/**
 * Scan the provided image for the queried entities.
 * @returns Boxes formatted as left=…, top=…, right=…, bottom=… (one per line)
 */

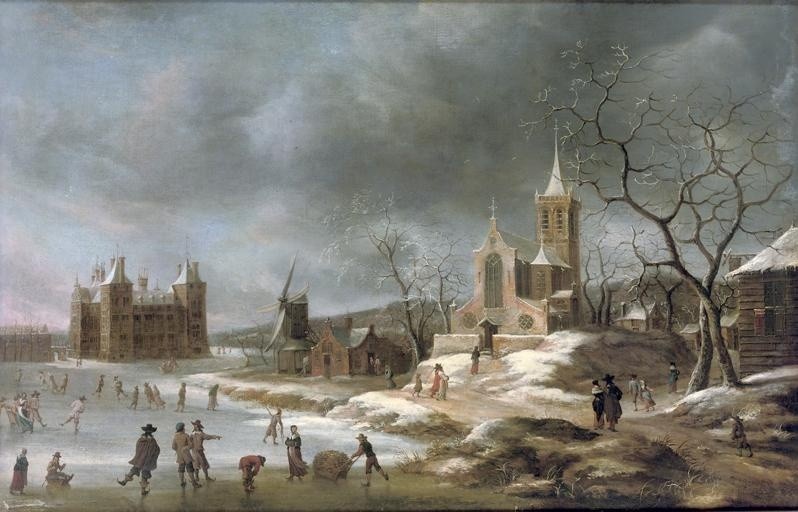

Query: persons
left=412, top=373, right=422, bottom=397
left=470, top=346, right=481, bottom=375
left=591, top=380, right=605, bottom=430
left=429, top=363, right=442, bottom=397
left=628, top=374, right=641, bottom=411
left=436, top=366, right=449, bottom=401
left=602, top=373, right=623, bottom=432
left=668, top=361, right=677, bottom=394
left=0, top=345, right=397, bottom=495
left=730, top=415, right=753, bottom=457
left=639, top=379, right=656, bottom=412
